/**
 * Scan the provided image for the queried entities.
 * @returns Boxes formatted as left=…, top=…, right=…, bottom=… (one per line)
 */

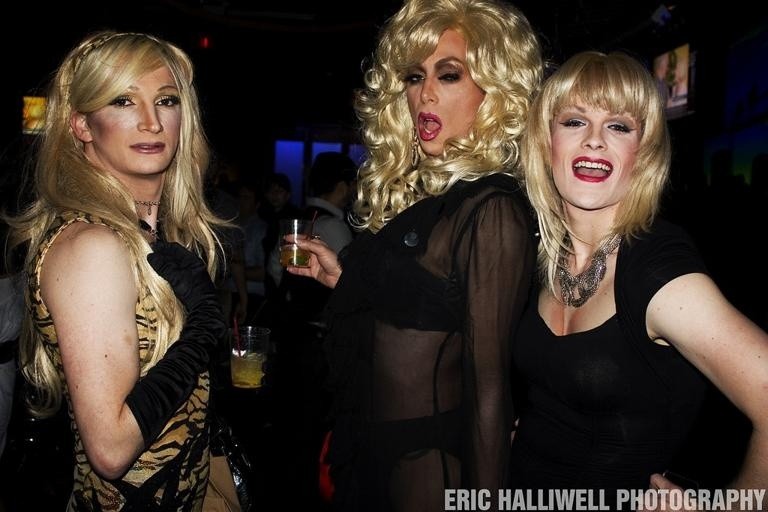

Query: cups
left=278, top=220, right=311, bottom=269
left=230, top=324, right=269, bottom=390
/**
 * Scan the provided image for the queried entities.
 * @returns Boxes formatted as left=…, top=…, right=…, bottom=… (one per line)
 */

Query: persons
left=2, top=0, right=767, bottom=512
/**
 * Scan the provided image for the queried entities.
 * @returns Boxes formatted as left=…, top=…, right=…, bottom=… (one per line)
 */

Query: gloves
left=122, top=240, right=233, bottom=453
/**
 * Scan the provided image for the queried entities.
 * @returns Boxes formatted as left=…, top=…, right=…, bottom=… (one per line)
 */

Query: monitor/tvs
left=652, top=41, right=696, bottom=120
